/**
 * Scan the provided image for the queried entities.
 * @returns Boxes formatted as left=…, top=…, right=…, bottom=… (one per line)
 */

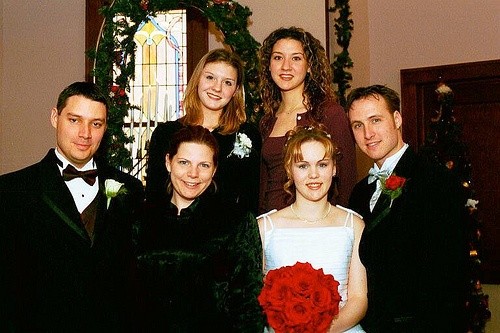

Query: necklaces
left=291, top=202, right=332, bottom=222
left=280, top=99, right=303, bottom=114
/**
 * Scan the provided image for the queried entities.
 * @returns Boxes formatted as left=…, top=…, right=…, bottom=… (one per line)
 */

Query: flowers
left=105, top=178, right=128, bottom=210
left=226, top=131, right=254, bottom=159
left=258, top=263, right=342, bottom=333
left=378, top=172, right=407, bottom=209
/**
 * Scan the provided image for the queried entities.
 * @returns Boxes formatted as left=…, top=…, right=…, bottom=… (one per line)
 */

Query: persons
left=147, top=49, right=262, bottom=223
left=256, top=124, right=368, bottom=333
left=119, top=126, right=262, bottom=333
left=348, top=85, right=486, bottom=333
left=258, top=27, right=359, bottom=208
left=0, top=81, right=146, bottom=333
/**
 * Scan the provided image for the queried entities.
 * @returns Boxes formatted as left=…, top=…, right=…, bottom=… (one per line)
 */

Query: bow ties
left=368, top=168, right=389, bottom=185
left=56, top=157, right=98, bottom=186
